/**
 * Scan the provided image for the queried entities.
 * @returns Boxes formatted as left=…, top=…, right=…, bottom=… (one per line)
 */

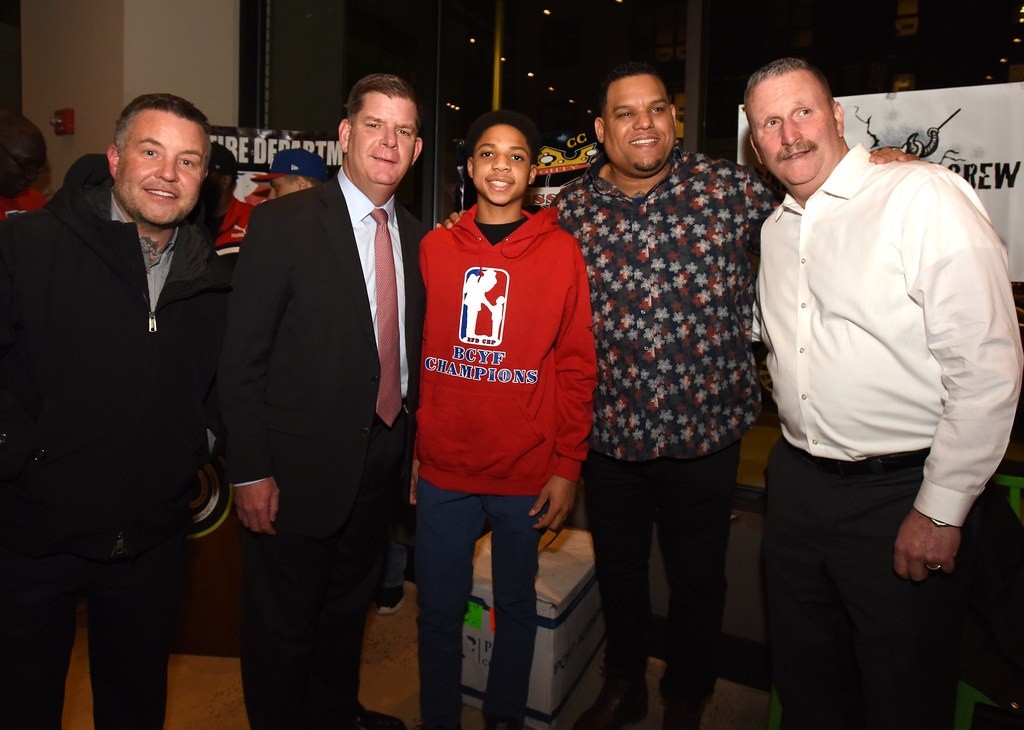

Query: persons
left=744, top=58, right=1023, bottom=730
left=251, top=147, right=407, bottom=614
left=412, top=111, right=597, bottom=730
left=207, top=74, right=428, bottom=730
left=0, top=108, right=255, bottom=272
left=433, top=62, right=919, bottom=730
left=0, top=93, right=232, bottom=730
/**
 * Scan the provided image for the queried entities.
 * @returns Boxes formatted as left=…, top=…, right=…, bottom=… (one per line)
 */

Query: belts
left=372, top=398, right=408, bottom=425
left=780, top=432, right=931, bottom=475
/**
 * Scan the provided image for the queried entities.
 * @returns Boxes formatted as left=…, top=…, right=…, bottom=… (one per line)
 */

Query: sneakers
left=373, top=584, right=405, bottom=615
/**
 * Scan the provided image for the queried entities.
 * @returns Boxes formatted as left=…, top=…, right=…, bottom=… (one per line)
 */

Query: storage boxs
left=461, top=529, right=610, bottom=730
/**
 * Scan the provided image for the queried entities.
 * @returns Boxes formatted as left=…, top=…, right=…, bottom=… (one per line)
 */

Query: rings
left=926, top=565, right=940, bottom=571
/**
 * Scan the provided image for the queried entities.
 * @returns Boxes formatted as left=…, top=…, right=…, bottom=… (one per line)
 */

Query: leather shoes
left=342, top=710, right=407, bottom=730
left=572, top=662, right=648, bottom=730
left=662, top=696, right=705, bottom=730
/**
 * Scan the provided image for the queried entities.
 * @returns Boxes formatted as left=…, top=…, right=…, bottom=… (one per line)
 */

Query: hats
left=252, top=148, right=328, bottom=183
left=208, top=141, right=237, bottom=172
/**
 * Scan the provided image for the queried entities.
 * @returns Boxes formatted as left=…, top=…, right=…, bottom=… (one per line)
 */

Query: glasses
left=0, top=144, right=46, bottom=172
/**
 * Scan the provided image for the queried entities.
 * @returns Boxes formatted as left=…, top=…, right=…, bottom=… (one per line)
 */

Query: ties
left=369, top=209, right=403, bottom=428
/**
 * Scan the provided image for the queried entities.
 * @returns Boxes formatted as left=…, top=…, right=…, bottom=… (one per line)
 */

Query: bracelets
left=931, top=519, right=946, bottom=526
